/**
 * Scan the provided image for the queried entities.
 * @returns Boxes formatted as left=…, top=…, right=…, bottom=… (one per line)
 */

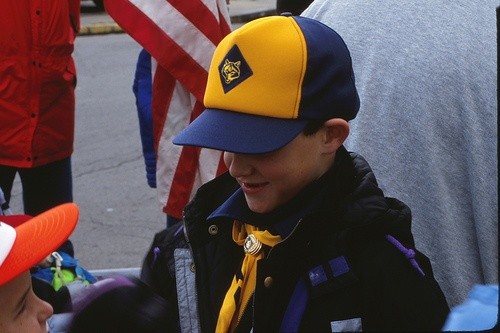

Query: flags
left=103, top=0, right=234, bottom=227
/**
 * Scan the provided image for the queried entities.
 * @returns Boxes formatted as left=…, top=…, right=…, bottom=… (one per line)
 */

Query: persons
left=139, top=12, right=450, bottom=333
left=0, top=203, right=79, bottom=333
left=0, top=0, right=80, bottom=258
left=300, top=0, right=500, bottom=307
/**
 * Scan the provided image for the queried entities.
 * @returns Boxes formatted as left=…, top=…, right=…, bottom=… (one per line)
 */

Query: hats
left=172, top=12, right=360, bottom=153
left=0, top=203, right=78, bottom=286
